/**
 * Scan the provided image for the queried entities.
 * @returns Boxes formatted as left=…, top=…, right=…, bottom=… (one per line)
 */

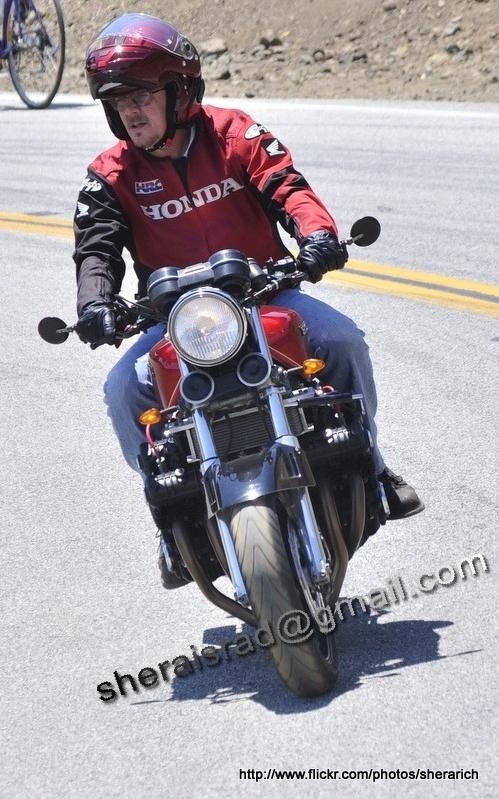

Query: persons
left=71, top=11, right=427, bottom=592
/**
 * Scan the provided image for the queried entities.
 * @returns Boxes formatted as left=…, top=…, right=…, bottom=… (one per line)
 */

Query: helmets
left=83, top=12, right=205, bottom=140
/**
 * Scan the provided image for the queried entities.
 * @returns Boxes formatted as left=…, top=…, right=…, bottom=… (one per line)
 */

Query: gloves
left=296, top=233, right=345, bottom=284
left=76, top=300, right=117, bottom=345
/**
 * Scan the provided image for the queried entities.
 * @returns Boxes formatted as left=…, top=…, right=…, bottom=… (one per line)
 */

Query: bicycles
left=1, top=0, right=68, bottom=112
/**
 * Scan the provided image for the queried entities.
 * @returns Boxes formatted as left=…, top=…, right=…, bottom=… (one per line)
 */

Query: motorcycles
left=37, top=217, right=381, bottom=698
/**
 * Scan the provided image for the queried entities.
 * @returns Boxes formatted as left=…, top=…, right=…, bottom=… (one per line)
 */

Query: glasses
left=109, top=87, right=167, bottom=112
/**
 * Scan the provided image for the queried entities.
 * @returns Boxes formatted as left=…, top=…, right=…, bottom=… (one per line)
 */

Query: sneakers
left=14, top=9, right=46, bottom=22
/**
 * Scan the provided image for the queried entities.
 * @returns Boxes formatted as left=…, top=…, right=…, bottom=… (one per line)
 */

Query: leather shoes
left=377, top=466, right=420, bottom=514
left=155, top=529, right=188, bottom=584
